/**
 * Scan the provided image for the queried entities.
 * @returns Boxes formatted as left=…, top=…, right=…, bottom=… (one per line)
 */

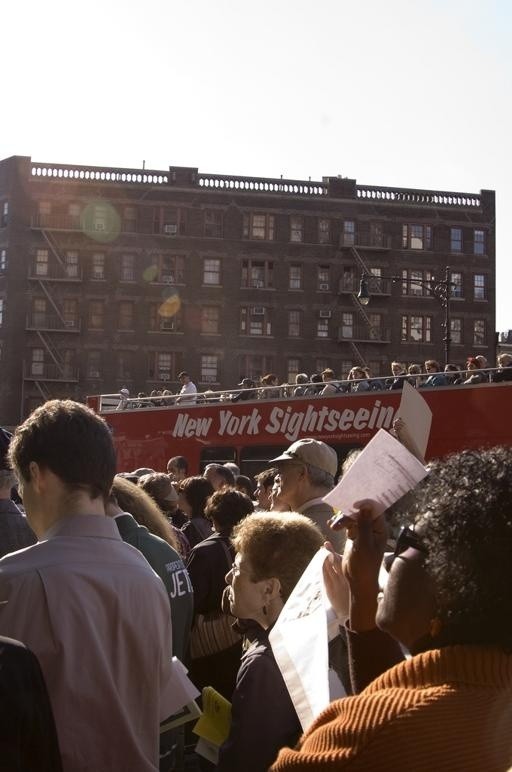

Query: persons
left=137, top=471, right=178, bottom=513
left=252, top=467, right=277, bottom=511
left=0, top=427, right=38, bottom=560
left=164, top=455, right=189, bottom=480
left=424, top=360, right=445, bottom=386
left=346, top=366, right=371, bottom=393
left=149, top=389, right=161, bottom=406
left=202, top=464, right=235, bottom=491
left=408, top=364, right=423, bottom=387
left=320, top=367, right=341, bottom=394
left=104, top=491, right=195, bottom=772
left=176, top=371, right=198, bottom=405
left=493, top=354, right=512, bottom=383
left=218, top=510, right=324, bottom=771
left=268, top=445, right=512, bottom=772
left=268, top=473, right=291, bottom=512
left=178, top=476, right=215, bottom=549
left=269, top=437, right=348, bottom=555
left=163, top=389, right=173, bottom=406
left=0, top=634, right=64, bottom=772
left=258, top=374, right=283, bottom=400
left=310, top=373, right=323, bottom=394
left=117, top=388, right=131, bottom=410
left=293, top=373, right=308, bottom=394
left=443, top=363, right=460, bottom=384
left=137, top=392, right=147, bottom=407
left=389, top=361, right=409, bottom=388
left=0, top=398, right=175, bottom=772
left=231, top=377, right=257, bottom=403
left=392, top=416, right=444, bottom=471
left=234, top=474, right=253, bottom=496
left=187, top=489, right=255, bottom=690
left=460, top=357, right=487, bottom=385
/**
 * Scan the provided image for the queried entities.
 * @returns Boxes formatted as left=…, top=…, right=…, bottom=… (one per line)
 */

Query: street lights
left=357, top=266, right=457, bottom=365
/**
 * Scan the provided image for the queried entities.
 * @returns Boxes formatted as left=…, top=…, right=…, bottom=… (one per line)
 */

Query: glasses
left=394, top=527, right=432, bottom=562
left=228, top=562, right=269, bottom=581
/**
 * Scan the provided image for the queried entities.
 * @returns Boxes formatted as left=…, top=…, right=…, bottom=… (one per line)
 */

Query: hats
left=1, top=427, right=17, bottom=471
left=118, top=387, right=131, bottom=396
left=267, top=436, right=338, bottom=477
left=177, top=371, right=190, bottom=378
left=237, top=378, right=253, bottom=386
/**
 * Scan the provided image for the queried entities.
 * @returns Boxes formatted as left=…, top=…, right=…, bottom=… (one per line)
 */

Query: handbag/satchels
left=188, top=539, right=244, bottom=662
